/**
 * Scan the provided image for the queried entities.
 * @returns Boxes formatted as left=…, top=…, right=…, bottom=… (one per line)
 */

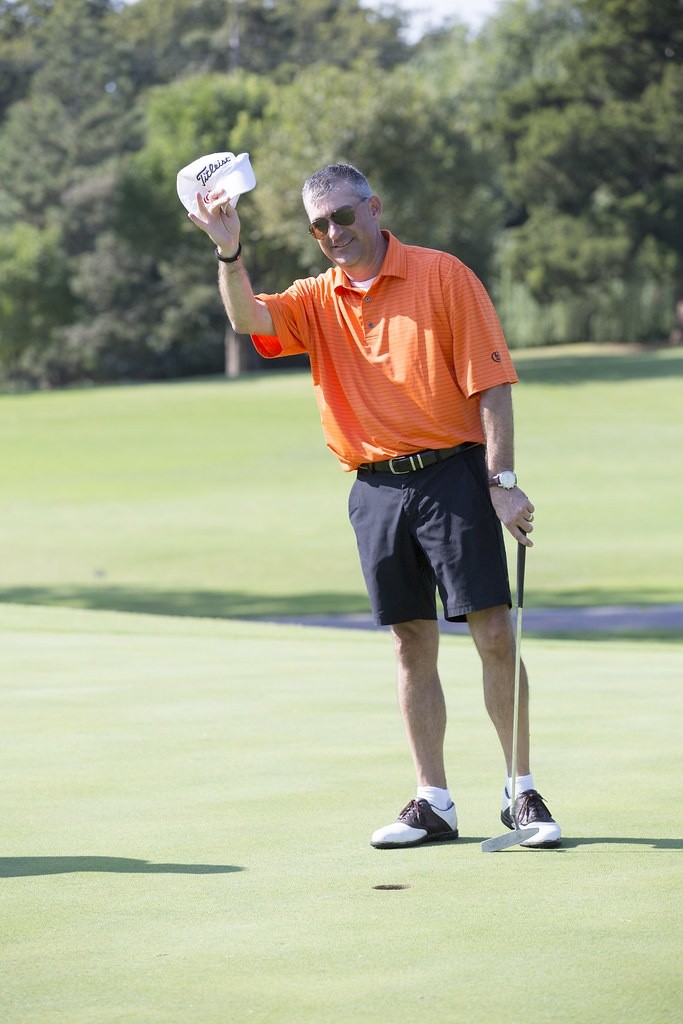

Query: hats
left=175, top=152, right=256, bottom=221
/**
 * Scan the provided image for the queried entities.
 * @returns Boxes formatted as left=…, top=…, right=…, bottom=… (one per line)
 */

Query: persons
left=188, top=163, right=560, bottom=848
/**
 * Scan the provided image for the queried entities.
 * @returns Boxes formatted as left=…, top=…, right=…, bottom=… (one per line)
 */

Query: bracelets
left=215, top=242, right=242, bottom=263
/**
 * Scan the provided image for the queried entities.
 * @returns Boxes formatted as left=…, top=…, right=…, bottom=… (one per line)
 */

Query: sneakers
left=501, top=786, right=562, bottom=848
left=369, top=796, right=459, bottom=849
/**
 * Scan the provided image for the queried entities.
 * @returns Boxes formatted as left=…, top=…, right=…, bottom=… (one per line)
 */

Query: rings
left=527, top=513, right=532, bottom=520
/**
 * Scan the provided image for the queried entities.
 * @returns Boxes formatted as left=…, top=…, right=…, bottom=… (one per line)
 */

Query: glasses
left=307, top=196, right=368, bottom=240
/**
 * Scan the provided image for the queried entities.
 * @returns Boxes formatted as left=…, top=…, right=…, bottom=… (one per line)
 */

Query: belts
left=358, top=441, right=481, bottom=475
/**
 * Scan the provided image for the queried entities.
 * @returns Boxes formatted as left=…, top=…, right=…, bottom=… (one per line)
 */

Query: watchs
left=488, top=471, right=518, bottom=490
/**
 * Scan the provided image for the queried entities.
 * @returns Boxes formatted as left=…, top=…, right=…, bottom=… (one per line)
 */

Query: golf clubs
left=480, top=525, right=540, bottom=853
